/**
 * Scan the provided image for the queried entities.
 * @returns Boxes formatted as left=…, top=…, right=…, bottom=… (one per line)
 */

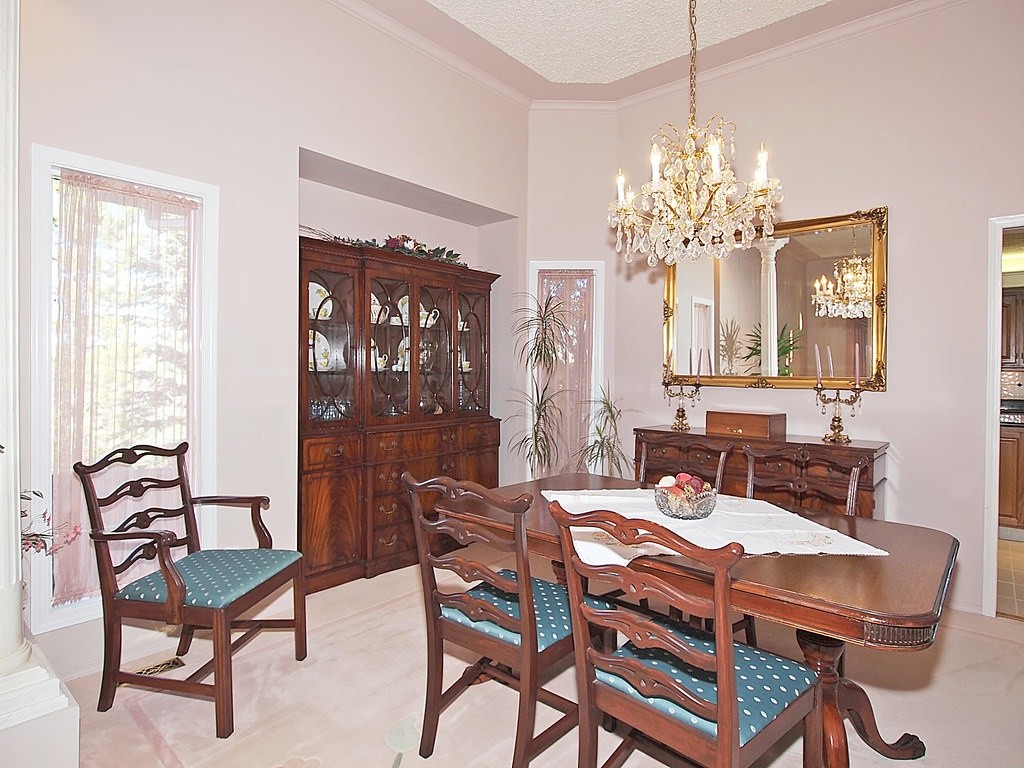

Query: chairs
left=401, top=434, right=869, bottom=768
left=73, top=442, right=307, bottom=738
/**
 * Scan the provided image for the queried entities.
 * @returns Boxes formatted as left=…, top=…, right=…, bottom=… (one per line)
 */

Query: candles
left=666, top=350, right=672, bottom=382
left=696, top=349, right=702, bottom=384
left=690, top=349, right=692, bottom=375
left=855, top=343, right=860, bottom=386
left=708, top=349, right=712, bottom=376
left=827, top=346, right=834, bottom=378
left=814, top=344, right=822, bottom=388
left=866, top=345, right=872, bottom=377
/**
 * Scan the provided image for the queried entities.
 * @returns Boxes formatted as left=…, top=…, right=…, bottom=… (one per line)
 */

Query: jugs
left=371, top=299, right=389, bottom=324
left=420, top=309, right=439, bottom=328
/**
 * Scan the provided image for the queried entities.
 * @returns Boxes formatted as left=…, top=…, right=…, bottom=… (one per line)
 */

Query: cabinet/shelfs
left=1002, top=287, right=1024, bottom=372
left=998, top=424, right=1024, bottom=542
left=298, top=235, right=502, bottom=595
left=633, top=425, right=890, bottom=521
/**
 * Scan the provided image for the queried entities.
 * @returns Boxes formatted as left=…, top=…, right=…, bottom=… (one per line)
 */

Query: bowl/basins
left=657, top=486, right=718, bottom=521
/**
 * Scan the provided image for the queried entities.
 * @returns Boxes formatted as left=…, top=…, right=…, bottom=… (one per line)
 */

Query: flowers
left=20, top=489, right=82, bottom=560
left=299, top=225, right=467, bottom=267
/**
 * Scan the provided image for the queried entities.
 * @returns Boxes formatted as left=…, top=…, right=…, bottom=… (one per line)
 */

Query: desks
left=434, top=472, right=960, bottom=768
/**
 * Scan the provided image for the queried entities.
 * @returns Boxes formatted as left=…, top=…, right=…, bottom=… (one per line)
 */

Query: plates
left=458, top=368, right=473, bottom=372
left=309, top=281, right=333, bottom=321
left=371, top=293, right=380, bottom=305
left=371, top=338, right=389, bottom=371
left=397, top=295, right=424, bottom=314
left=309, top=330, right=333, bottom=371
left=398, top=337, right=409, bottom=363
left=458, top=309, right=470, bottom=331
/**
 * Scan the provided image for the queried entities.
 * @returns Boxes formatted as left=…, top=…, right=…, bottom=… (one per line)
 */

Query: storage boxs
left=706, top=411, right=786, bottom=438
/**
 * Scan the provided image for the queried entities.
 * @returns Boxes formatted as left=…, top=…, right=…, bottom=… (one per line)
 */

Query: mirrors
left=663, top=207, right=889, bottom=392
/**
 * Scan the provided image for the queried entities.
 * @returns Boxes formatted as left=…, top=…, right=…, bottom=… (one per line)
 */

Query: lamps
left=607, top=0, right=784, bottom=267
left=810, top=230, right=872, bottom=319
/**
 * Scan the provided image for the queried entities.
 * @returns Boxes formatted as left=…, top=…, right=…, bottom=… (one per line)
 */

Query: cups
left=459, top=322, right=468, bottom=328
left=462, top=362, right=470, bottom=368
left=390, top=315, right=402, bottom=327
left=377, top=354, right=388, bottom=368
left=316, top=359, right=328, bottom=366
left=313, top=308, right=329, bottom=317
